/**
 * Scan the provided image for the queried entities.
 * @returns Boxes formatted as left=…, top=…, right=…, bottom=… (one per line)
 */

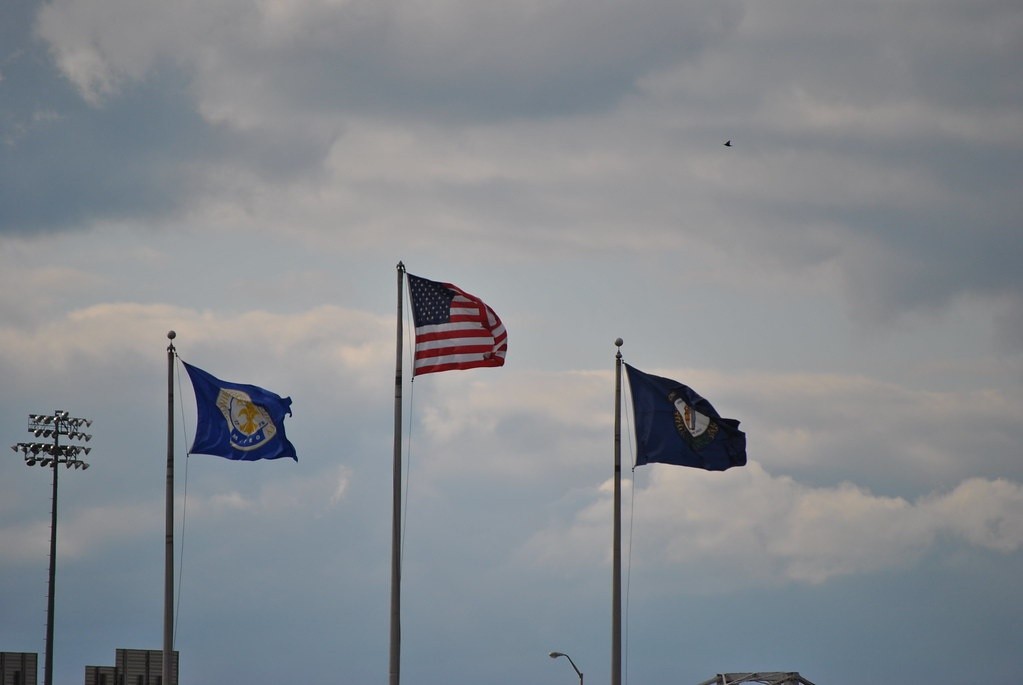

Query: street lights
left=12, top=411, right=92, bottom=685
left=548, top=652, right=583, bottom=685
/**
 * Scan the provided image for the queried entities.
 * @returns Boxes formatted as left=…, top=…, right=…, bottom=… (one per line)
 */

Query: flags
left=624, top=362, right=747, bottom=470
left=406, top=273, right=507, bottom=376
left=182, top=360, right=299, bottom=463
